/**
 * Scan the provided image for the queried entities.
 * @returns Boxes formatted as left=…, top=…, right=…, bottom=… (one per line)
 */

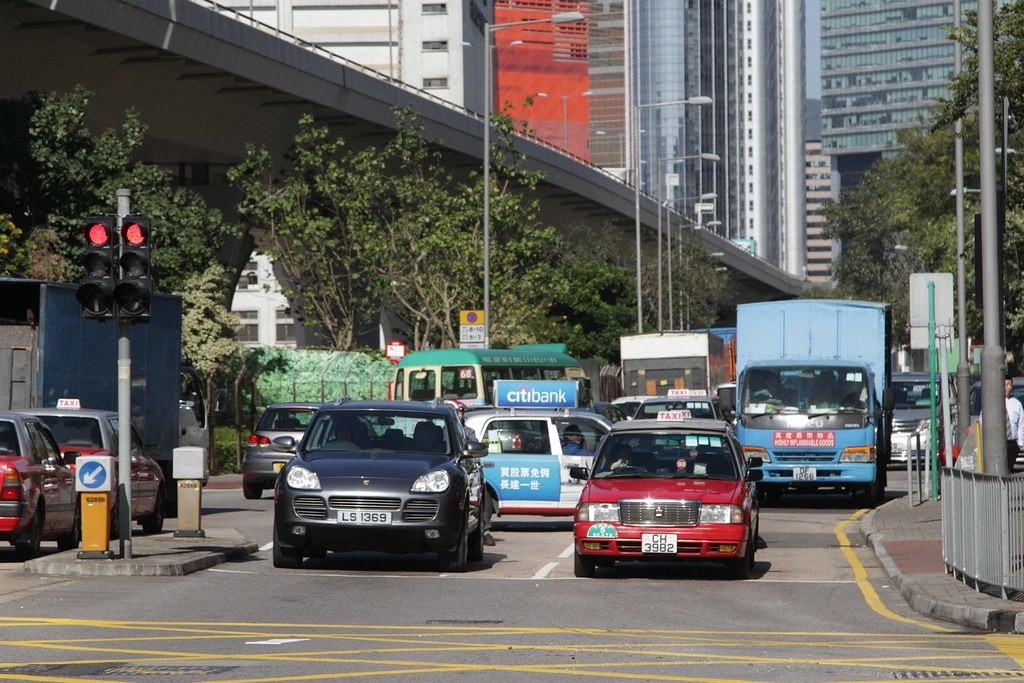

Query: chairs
left=341, top=416, right=363, bottom=443
left=921, top=388, right=930, bottom=399
left=380, top=428, right=405, bottom=448
left=275, top=411, right=295, bottom=427
left=411, top=421, right=436, bottom=445
left=414, top=425, right=448, bottom=451
left=897, top=389, right=908, bottom=401
left=687, top=444, right=729, bottom=471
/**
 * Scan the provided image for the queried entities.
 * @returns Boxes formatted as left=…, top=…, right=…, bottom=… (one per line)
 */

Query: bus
left=391, top=342, right=592, bottom=436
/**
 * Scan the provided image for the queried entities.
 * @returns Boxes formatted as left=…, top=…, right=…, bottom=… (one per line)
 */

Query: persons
left=562, top=425, right=596, bottom=455
left=980, top=374, right=1024, bottom=475
left=442, top=407, right=478, bottom=449
left=597, top=443, right=637, bottom=474
left=749, top=372, right=786, bottom=405
left=801, top=367, right=880, bottom=411
left=325, top=422, right=365, bottom=451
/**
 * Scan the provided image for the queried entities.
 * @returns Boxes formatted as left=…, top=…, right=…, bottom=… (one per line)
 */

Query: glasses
left=576, top=435, right=580, bottom=437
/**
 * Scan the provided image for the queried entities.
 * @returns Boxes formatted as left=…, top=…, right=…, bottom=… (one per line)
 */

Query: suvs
left=273, top=399, right=490, bottom=567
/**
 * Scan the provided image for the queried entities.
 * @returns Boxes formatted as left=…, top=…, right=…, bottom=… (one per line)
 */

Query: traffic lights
left=77, top=215, right=153, bottom=324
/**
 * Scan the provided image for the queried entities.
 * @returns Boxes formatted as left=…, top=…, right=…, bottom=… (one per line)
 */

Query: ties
left=1006, top=409, right=1012, bottom=440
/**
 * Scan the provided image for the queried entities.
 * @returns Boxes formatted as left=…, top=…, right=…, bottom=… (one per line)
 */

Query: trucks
left=0, top=276, right=213, bottom=515
left=729, top=298, right=894, bottom=500
left=619, top=330, right=725, bottom=414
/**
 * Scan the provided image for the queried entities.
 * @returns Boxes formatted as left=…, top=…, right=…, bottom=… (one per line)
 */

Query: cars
left=592, top=393, right=734, bottom=449
left=969, top=380, right=1024, bottom=419
left=890, top=372, right=958, bottom=469
left=0, top=397, right=165, bottom=562
left=462, top=407, right=631, bottom=521
left=242, top=404, right=334, bottom=498
left=570, top=416, right=764, bottom=574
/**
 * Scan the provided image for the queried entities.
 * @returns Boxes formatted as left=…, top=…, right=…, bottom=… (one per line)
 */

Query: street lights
left=537, top=90, right=592, bottom=151
left=597, top=129, right=645, bottom=181
left=458, top=12, right=588, bottom=344
left=631, top=98, right=729, bottom=332
